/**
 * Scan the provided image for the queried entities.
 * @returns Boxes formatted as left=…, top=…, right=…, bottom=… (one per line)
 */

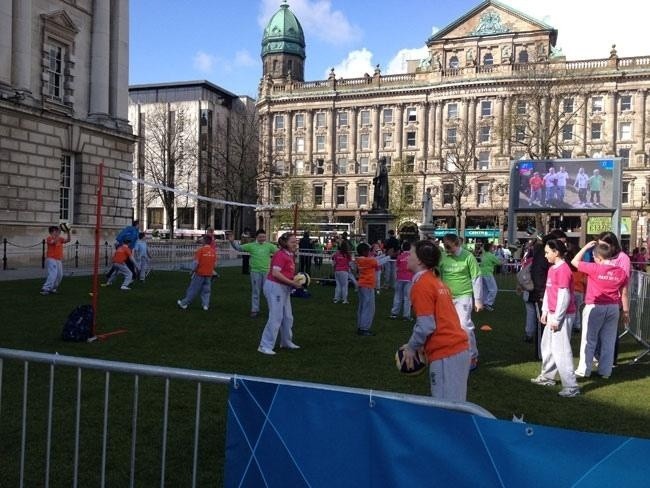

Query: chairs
left=121, top=286, right=130, bottom=289
left=203, top=305, right=208, bottom=311
left=257, top=346, right=276, bottom=356
left=280, top=340, right=300, bottom=350
left=333, top=298, right=413, bottom=337
left=470, top=304, right=609, bottom=398
left=177, top=300, right=188, bottom=309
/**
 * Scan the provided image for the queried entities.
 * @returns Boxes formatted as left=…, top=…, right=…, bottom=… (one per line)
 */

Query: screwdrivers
left=293, top=272, right=310, bottom=288
left=395, top=344, right=427, bottom=375
left=60, top=222, right=71, bottom=231
left=375, top=248, right=382, bottom=255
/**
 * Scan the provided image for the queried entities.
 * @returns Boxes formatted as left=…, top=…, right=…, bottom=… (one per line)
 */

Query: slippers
left=61, top=305, right=93, bottom=342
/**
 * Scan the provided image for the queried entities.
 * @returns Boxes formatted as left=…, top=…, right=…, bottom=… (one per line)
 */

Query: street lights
left=517, top=261, right=534, bottom=291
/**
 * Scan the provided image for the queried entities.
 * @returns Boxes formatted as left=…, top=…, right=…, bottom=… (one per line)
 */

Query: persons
left=461, top=234, right=515, bottom=312
left=202, top=227, right=219, bottom=283
left=240, top=227, right=251, bottom=275
left=257, top=231, right=301, bottom=355
left=103, top=220, right=154, bottom=290
left=569, top=239, right=630, bottom=382
left=519, top=230, right=633, bottom=371
left=298, top=228, right=413, bottom=336
left=439, top=233, right=483, bottom=373
left=530, top=240, right=581, bottom=399
left=517, top=163, right=605, bottom=208
left=178, top=236, right=215, bottom=311
left=421, top=188, right=433, bottom=224
left=227, top=229, right=280, bottom=317
left=633, top=238, right=650, bottom=271
left=372, top=155, right=390, bottom=212
left=399, top=239, right=470, bottom=404
left=41, top=224, right=71, bottom=296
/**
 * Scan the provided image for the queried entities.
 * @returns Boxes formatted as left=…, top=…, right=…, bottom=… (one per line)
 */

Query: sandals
left=143, top=227, right=234, bottom=241
left=276, top=222, right=353, bottom=245
